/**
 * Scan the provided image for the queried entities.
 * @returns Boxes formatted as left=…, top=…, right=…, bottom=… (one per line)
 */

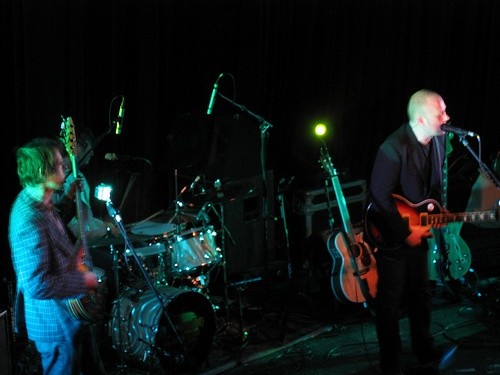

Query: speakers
left=0, top=309, right=28, bottom=375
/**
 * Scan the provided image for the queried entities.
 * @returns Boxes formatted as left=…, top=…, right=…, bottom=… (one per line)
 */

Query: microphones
left=190, top=174, right=203, bottom=188
left=105, top=152, right=144, bottom=161
left=115, top=97, right=125, bottom=135
left=440, top=124, right=478, bottom=137
left=207, top=72, right=224, bottom=115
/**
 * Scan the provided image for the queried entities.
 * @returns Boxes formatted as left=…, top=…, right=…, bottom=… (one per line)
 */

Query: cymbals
left=86, top=234, right=148, bottom=248
left=188, top=176, right=263, bottom=207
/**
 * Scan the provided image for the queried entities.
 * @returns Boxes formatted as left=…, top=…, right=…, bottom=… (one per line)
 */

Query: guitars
left=316, top=151, right=379, bottom=305
left=59, top=116, right=119, bottom=324
left=426, top=133, right=472, bottom=281
left=363, top=193, right=500, bottom=250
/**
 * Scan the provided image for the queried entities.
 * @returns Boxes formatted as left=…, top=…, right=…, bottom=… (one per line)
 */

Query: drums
left=112, top=284, right=216, bottom=366
left=129, top=216, right=187, bottom=245
left=166, top=225, right=223, bottom=278
left=117, top=243, right=167, bottom=284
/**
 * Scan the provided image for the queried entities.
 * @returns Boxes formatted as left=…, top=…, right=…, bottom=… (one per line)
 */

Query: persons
left=370, top=90, right=450, bottom=375
left=8, top=138, right=99, bottom=375
